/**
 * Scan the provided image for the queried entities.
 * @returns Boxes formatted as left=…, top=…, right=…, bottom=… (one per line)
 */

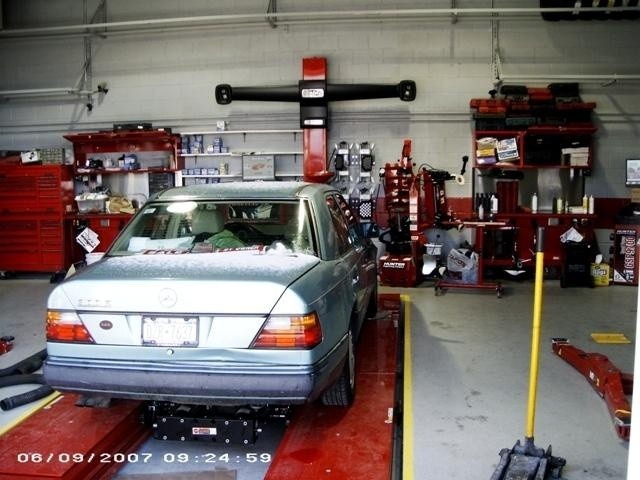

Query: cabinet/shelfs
left=471, top=102, right=597, bottom=279
left=63, top=131, right=182, bottom=262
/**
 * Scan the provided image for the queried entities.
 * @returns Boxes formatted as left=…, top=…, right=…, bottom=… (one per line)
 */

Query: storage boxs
left=0, top=163, right=84, bottom=272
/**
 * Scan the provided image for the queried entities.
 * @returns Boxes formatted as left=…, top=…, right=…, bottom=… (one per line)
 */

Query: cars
left=43, top=182, right=377, bottom=408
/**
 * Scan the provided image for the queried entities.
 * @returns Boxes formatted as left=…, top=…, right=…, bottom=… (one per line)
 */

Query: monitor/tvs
left=624, top=158, right=640, bottom=188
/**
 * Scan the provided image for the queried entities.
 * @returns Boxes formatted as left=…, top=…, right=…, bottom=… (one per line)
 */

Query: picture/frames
left=242, top=154, right=276, bottom=181
